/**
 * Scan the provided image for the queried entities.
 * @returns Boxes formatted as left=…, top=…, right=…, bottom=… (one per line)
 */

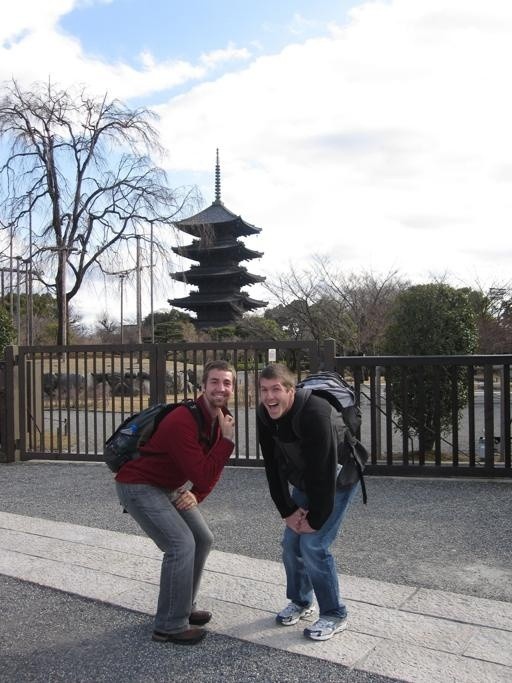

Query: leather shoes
left=190, top=611, right=212, bottom=625
left=152, top=628, right=205, bottom=645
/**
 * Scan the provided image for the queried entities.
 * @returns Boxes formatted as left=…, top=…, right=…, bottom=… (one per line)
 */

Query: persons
left=116, top=360, right=240, bottom=646
left=255, top=362, right=360, bottom=641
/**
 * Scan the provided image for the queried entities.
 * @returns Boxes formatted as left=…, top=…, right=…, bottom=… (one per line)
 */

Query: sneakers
left=304, top=617, right=348, bottom=641
left=277, top=603, right=315, bottom=626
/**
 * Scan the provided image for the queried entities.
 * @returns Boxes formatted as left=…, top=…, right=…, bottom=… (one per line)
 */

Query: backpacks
left=105, top=399, right=205, bottom=472
left=296, top=370, right=361, bottom=441
